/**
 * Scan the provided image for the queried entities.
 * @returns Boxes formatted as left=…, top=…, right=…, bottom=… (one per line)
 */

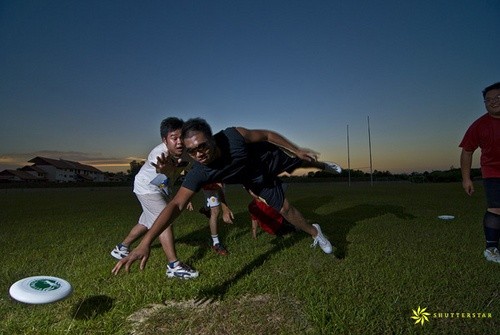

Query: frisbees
left=438, top=215, right=455, bottom=220
left=10, top=275, right=71, bottom=305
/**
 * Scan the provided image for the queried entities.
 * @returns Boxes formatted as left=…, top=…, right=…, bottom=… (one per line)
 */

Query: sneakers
left=166, top=258, right=200, bottom=280
left=323, top=161, right=343, bottom=174
left=210, top=242, right=229, bottom=256
left=309, top=223, right=333, bottom=255
left=483, top=247, right=500, bottom=263
left=110, top=243, right=130, bottom=260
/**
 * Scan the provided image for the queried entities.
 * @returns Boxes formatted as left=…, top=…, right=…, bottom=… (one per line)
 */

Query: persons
left=199, top=182, right=234, bottom=255
left=459, top=83, right=500, bottom=264
left=110, top=118, right=199, bottom=280
left=244, top=184, right=301, bottom=242
left=111, top=117, right=343, bottom=276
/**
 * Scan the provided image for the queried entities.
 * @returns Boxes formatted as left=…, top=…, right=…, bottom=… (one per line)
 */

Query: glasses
left=186, top=141, right=209, bottom=156
